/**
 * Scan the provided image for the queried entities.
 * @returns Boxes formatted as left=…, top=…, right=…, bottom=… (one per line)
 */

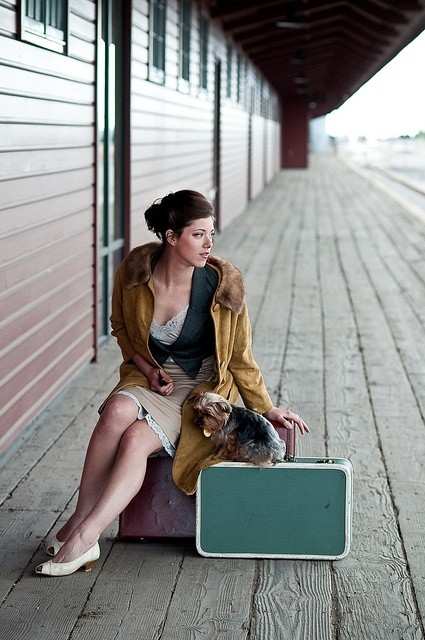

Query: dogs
left=185, top=392, right=287, bottom=471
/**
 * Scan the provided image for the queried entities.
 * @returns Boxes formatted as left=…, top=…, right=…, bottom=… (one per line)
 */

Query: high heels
left=44, top=534, right=65, bottom=556
left=35, top=541, right=101, bottom=578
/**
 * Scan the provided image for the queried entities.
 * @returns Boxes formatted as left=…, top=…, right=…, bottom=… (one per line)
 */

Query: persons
left=35, top=190, right=310, bottom=576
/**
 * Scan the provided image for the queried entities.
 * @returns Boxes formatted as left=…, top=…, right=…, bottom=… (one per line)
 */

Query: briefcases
left=192, top=455, right=354, bottom=561
